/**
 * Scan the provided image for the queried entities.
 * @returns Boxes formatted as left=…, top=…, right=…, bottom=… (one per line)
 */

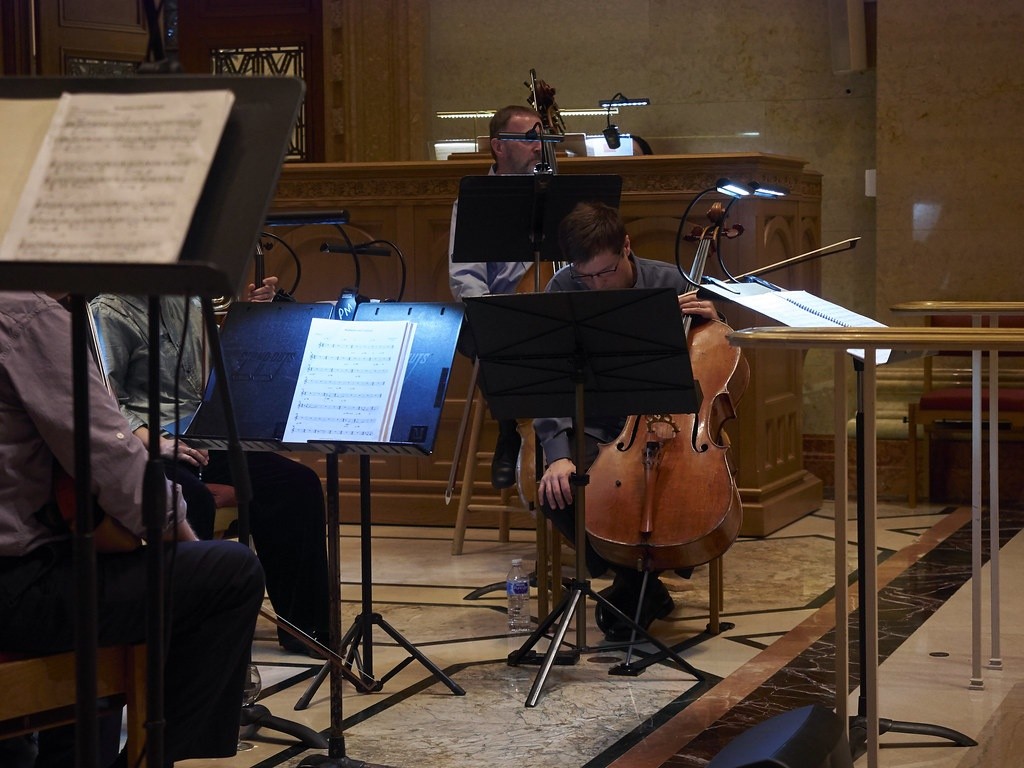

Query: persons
left=0, top=288, right=265, bottom=767
left=448, top=106, right=575, bottom=491
left=90, top=277, right=341, bottom=656
left=527, top=205, right=720, bottom=642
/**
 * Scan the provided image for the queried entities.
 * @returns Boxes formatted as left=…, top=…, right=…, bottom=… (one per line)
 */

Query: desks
left=727, top=324, right=1024, bottom=768
left=887, top=301, right=1024, bottom=691
left=240, top=151, right=824, bottom=538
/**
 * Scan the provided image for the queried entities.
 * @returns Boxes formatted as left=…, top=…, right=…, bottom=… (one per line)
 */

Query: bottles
left=506, top=558, right=531, bottom=634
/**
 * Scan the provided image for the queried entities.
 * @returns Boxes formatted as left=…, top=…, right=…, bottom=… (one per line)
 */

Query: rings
left=186, top=448, right=191, bottom=454
left=697, top=301, right=700, bottom=307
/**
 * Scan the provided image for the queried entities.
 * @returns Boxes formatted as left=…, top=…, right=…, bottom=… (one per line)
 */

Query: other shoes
left=285, top=629, right=334, bottom=659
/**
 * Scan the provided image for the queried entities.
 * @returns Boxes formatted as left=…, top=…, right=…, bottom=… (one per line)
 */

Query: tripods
left=292, top=282, right=978, bottom=767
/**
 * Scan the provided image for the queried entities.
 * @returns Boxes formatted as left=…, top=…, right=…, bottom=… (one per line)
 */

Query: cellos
left=580, top=198, right=746, bottom=679
left=514, top=64, right=580, bottom=551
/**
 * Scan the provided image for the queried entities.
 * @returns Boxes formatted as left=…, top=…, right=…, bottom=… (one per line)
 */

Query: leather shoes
left=595, top=577, right=675, bottom=642
left=490, top=431, right=544, bottom=489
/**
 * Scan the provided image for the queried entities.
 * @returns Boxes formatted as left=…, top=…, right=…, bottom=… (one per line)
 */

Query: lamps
left=675, top=178, right=751, bottom=295
left=716, top=181, right=791, bottom=292
left=598, top=92, right=651, bottom=149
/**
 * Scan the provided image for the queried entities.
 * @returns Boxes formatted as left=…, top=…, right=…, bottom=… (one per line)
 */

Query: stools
left=0, top=644, right=147, bottom=768
left=452, top=385, right=537, bottom=555
left=536, top=494, right=724, bottom=637
left=205, top=483, right=239, bottom=542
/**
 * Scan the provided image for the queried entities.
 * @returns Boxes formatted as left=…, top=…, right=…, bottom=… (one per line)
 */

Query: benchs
left=903, top=315, right=1024, bottom=510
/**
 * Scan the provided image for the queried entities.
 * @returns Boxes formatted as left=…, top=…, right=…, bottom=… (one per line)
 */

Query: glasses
left=570, top=249, right=622, bottom=283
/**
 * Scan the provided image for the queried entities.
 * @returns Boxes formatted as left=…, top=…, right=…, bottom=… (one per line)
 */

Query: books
left=0, top=88, right=235, bottom=263
left=739, top=288, right=893, bottom=365
